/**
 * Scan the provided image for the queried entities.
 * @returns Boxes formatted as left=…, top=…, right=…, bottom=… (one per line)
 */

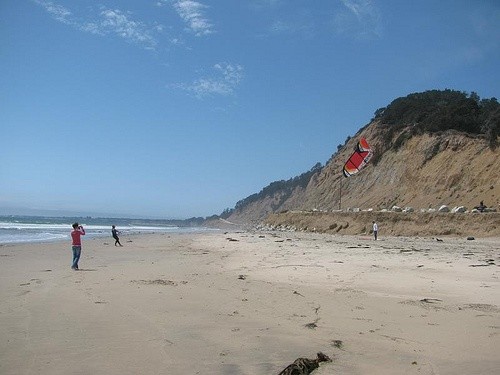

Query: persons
left=112, top=225, right=123, bottom=246
left=480, top=199, right=484, bottom=213
left=372, top=221, right=378, bottom=240
left=70, top=222, right=85, bottom=270
